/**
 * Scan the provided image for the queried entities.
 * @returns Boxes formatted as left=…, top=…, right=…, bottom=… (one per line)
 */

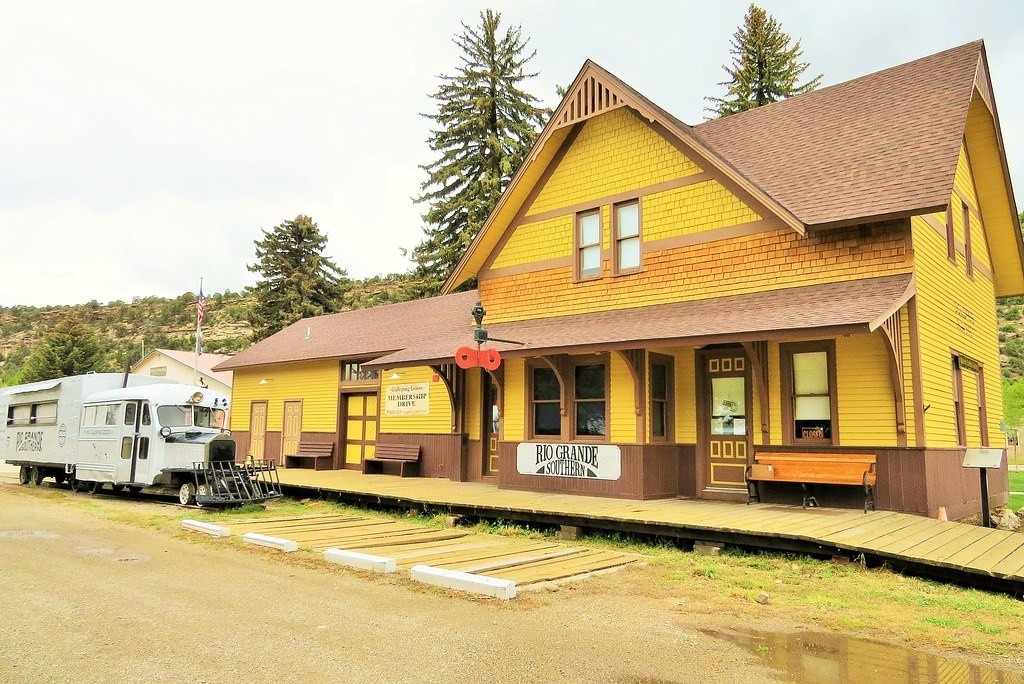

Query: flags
left=198, top=333, right=202, bottom=356
left=198, top=293, right=205, bottom=324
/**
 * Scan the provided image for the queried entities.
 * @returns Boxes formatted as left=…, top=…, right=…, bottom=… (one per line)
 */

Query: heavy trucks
left=0, top=371, right=284, bottom=513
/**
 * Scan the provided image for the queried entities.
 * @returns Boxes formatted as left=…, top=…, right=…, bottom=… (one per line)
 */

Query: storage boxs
left=752, top=464, right=775, bottom=480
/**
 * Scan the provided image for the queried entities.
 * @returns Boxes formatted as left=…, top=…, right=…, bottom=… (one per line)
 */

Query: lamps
left=259, top=379, right=274, bottom=385
left=390, top=372, right=406, bottom=379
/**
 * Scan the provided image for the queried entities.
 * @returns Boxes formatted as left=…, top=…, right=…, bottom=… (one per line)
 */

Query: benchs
left=284, top=440, right=334, bottom=471
left=361, top=442, right=421, bottom=478
left=745, top=451, right=878, bottom=514
